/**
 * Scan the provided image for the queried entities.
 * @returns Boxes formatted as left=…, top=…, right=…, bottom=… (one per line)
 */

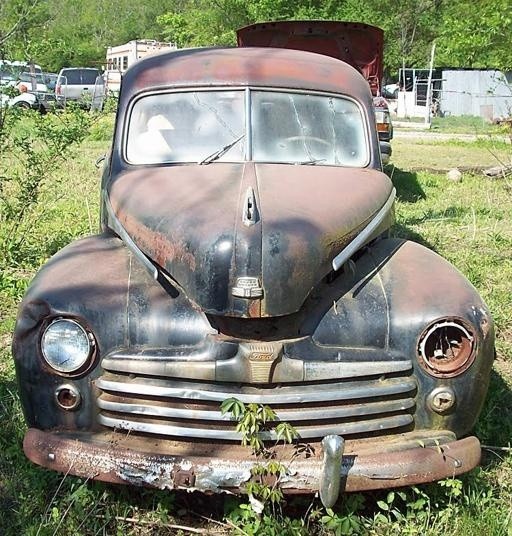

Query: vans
left=0, top=59, right=104, bottom=115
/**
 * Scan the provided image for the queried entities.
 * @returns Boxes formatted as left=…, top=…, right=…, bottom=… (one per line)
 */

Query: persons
left=432, top=99, right=439, bottom=114
left=46, top=78, right=55, bottom=92
